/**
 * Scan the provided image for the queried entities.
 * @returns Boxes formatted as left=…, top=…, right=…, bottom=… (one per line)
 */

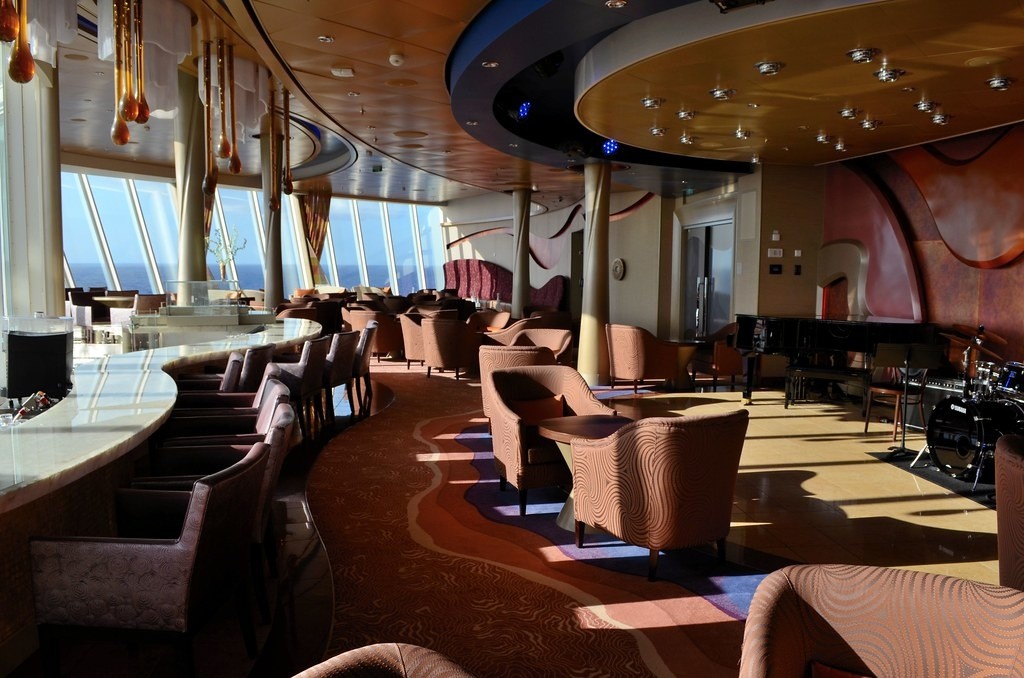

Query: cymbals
left=952, top=323, right=1008, bottom=347
left=940, top=332, right=1004, bottom=363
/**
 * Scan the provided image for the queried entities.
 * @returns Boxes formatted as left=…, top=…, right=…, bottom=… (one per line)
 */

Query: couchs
left=464, top=302, right=578, bottom=436
left=570, top=409, right=751, bottom=581
left=689, top=321, right=741, bottom=393
left=23, top=286, right=477, bottom=678
left=489, top=364, right=617, bottom=518
left=739, top=564, right=1024, bottom=676
left=604, top=324, right=680, bottom=394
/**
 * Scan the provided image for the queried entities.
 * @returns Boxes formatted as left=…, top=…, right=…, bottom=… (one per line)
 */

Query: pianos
left=725, top=313, right=942, bottom=407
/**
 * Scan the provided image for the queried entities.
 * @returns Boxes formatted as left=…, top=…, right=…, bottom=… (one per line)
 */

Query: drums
left=926, top=397, right=1017, bottom=479
left=971, top=360, right=1002, bottom=386
left=995, top=360, right=1024, bottom=393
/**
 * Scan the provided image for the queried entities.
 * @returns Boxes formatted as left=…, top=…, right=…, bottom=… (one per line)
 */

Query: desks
left=536, top=414, right=639, bottom=536
left=662, top=335, right=711, bottom=393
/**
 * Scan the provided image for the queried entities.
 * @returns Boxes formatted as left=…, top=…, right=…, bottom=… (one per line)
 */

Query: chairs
left=864, top=365, right=931, bottom=443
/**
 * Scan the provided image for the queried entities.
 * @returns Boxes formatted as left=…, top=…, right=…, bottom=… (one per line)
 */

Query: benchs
left=783, top=364, right=873, bottom=418
